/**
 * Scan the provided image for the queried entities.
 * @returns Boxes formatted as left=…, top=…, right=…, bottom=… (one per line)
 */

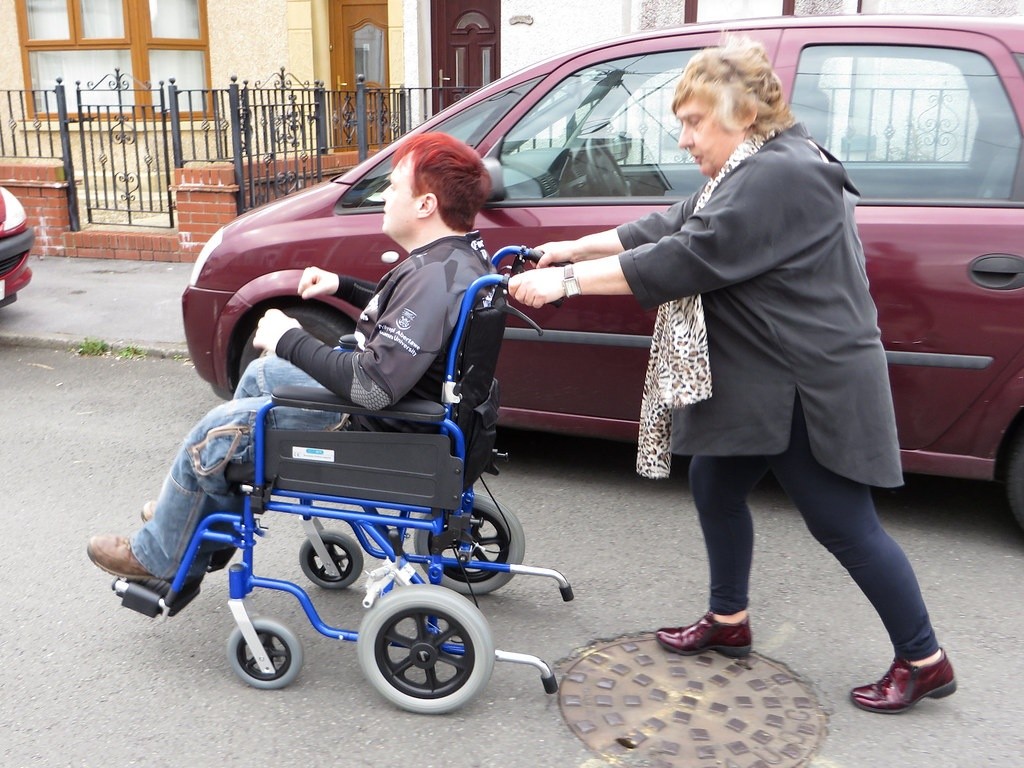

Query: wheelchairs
left=111, top=243, right=574, bottom=715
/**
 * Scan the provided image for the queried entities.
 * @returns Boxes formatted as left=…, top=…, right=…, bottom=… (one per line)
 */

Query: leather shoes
left=851, top=646, right=957, bottom=714
left=656, top=611, right=750, bottom=656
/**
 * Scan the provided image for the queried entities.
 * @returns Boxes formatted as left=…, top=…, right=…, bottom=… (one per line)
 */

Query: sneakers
left=87, top=534, right=159, bottom=580
left=141, top=501, right=156, bottom=521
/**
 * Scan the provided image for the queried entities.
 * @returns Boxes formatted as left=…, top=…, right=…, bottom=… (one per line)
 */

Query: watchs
left=560, top=264, right=582, bottom=297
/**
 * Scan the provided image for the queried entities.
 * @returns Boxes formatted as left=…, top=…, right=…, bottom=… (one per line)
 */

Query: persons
left=85, top=130, right=499, bottom=585
left=505, top=28, right=961, bottom=714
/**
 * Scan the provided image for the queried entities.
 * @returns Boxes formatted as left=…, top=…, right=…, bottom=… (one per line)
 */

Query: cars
left=182, top=15, right=1024, bottom=529
left=0, top=186, right=34, bottom=308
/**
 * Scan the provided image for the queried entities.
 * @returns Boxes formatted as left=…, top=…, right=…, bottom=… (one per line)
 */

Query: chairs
left=794, top=87, right=827, bottom=146
left=976, top=83, right=1020, bottom=200
